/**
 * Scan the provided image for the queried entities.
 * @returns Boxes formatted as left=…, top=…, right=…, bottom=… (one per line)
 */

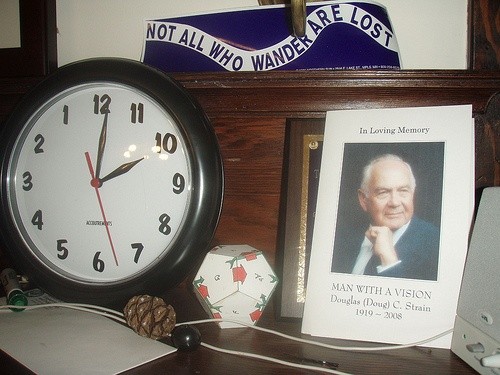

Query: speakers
left=450, top=187, right=499, bottom=375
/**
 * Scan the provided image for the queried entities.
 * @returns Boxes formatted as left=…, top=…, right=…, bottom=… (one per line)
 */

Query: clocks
left=5, top=58, right=224, bottom=309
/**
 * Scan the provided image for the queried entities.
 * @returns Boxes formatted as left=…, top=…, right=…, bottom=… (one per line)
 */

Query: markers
left=1, top=259, right=28, bottom=311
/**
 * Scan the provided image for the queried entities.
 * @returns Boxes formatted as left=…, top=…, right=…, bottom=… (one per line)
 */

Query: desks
left=2, top=281, right=479, bottom=374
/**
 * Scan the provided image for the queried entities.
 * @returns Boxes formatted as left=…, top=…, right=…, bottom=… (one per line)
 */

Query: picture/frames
left=278, top=119, right=326, bottom=318
